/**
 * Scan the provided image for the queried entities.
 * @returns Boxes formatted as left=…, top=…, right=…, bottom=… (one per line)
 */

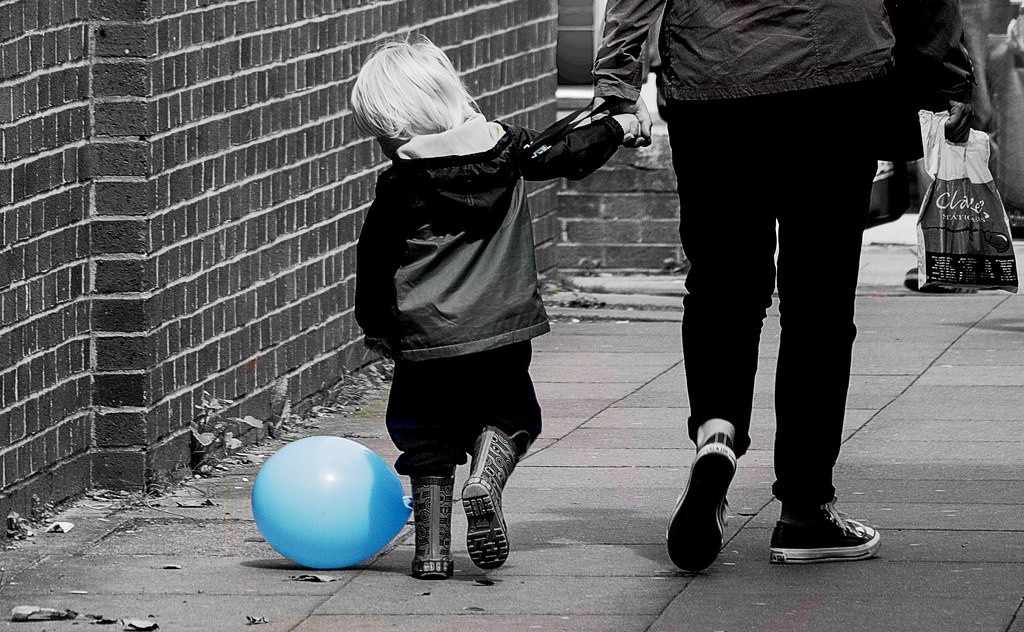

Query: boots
left=409, top=475, right=455, bottom=580
left=461, top=426, right=510, bottom=571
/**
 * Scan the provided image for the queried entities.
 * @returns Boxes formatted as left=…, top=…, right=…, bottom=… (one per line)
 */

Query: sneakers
left=664, top=432, right=737, bottom=572
left=769, top=495, right=882, bottom=565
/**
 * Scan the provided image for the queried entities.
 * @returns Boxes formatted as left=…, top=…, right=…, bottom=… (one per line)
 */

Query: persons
left=350, top=43, right=642, bottom=578
left=591, top=0, right=977, bottom=571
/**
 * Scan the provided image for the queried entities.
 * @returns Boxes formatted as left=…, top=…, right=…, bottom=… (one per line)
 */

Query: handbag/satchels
left=917, top=107, right=1019, bottom=296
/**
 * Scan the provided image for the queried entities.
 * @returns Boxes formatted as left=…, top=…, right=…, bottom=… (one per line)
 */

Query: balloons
left=251, top=436, right=410, bottom=568
